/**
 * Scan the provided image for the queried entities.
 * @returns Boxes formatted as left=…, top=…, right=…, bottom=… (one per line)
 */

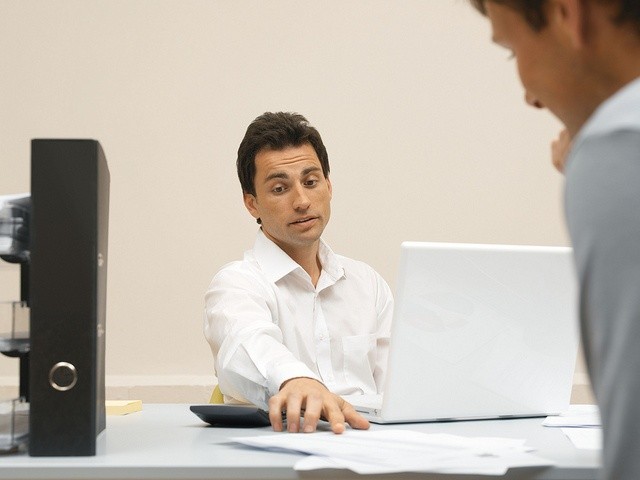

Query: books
left=105, top=400, right=142, bottom=416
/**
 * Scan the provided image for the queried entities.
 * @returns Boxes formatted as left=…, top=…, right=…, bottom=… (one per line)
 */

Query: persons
left=204, top=112, right=395, bottom=434
left=468, top=0, right=640, bottom=480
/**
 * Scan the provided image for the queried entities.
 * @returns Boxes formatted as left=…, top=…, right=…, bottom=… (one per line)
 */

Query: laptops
left=336, top=239, right=581, bottom=426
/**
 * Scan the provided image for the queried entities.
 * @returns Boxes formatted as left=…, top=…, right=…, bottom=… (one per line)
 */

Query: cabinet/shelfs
left=0, top=197, right=31, bottom=455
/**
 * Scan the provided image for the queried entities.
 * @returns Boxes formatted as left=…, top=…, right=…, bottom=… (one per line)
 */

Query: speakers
left=29, top=133, right=111, bottom=454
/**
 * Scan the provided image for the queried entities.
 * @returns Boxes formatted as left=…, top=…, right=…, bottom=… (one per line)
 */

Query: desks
left=0, top=402, right=601, bottom=480
